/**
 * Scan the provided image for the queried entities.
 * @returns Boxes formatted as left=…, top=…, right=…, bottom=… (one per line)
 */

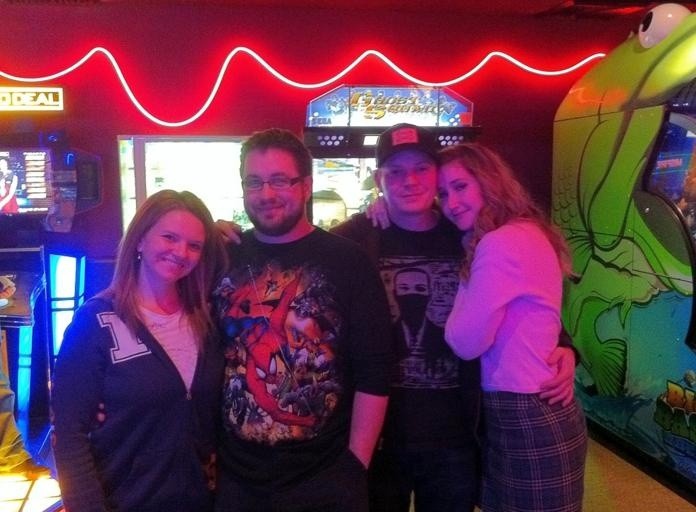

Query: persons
left=213, top=121, right=581, bottom=511
left=364, top=143, right=589, bottom=512
left=48, top=188, right=243, bottom=510
left=0, top=357, right=52, bottom=486
left=97, top=127, right=400, bottom=512
left=385, top=266, right=463, bottom=389
left=0, top=156, right=21, bottom=216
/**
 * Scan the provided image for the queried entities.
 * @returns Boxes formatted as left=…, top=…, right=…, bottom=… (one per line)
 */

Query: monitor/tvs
left=0, top=148, right=54, bottom=218
left=133, top=135, right=255, bottom=235
left=306, top=146, right=383, bottom=234
left=117, top=134, right=136, bottom=235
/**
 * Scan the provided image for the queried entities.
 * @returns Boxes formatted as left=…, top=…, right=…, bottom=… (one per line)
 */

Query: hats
left=376, top=122, right=441, bottom=168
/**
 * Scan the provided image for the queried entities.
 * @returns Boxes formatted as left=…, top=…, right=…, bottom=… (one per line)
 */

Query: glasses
left=239, top=175, right=303, bottom=190
